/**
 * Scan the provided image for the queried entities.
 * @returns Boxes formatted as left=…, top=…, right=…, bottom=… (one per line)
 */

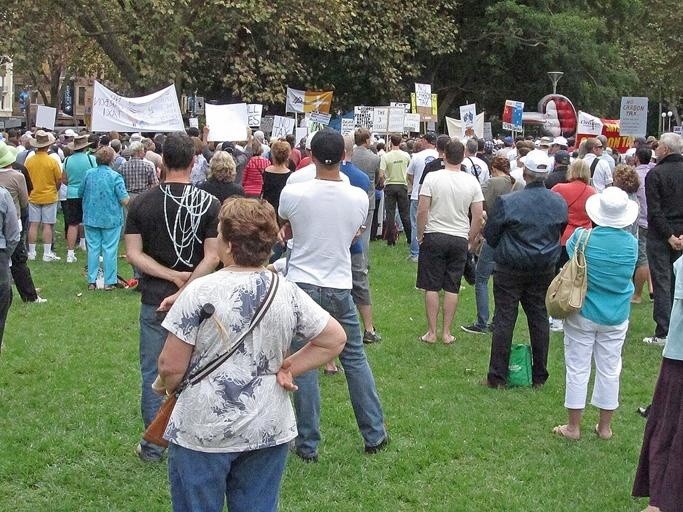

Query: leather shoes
left=638, top=404, right=651, bottom=417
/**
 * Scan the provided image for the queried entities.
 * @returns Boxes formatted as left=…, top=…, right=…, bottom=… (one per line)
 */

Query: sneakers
left=643, top=336, right=667, bottom=346
left=136, top=443, right=161, bottom=462
left=407, top=254, right=419, bottom=263
left=28, top=288, right=47, bottom=302
left=532, top=383, right=543, bottom=389
left=289, top=440, right=317, bottom=463
left=549, top=315, right=563, bottom=334
left=363, top=326, right=381, bottom=343
left=88, top=283, right=113, bottom=290
left=324, top=365, right=342, bottom=374
left=370, top=231, right=411, bottom=246
left=479, top=379, right=505, bottom=389
left=630, top=293, right=654, bottom=304
left=365, top=435, right=390, bottom=453
left=461, top=324, right=494, bottom=334
left=28, top=246, right=86, bottom=263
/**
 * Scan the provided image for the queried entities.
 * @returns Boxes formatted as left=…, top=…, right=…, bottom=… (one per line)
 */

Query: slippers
left=416, top=334, right=437, bottom=343
left=552, top=424, right=580, bottom=441
left=442, top=335, right=456, bottom=344
left=595, top=423, right=612, bottom=439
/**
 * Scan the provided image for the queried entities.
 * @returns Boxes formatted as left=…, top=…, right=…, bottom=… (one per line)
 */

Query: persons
left=276, top=127, right=390, bottom=462
left=536, top=133, right=683, bottom=344
left=631, top=253, right=683, bottom=512
left=480, top=149, right=569, bottom=389
left=189, top=127, right=311, bottom=209
left=342, top=127, right=536, bottom=345
left=151, top=194, right=348, bottom=512
left=0, top=125, right=167, bottom=343
left=285, top=163, right=351, bottom=376
left=125, top=129, right=221, bottom=464
left=553, top=186, right=639, bottom=438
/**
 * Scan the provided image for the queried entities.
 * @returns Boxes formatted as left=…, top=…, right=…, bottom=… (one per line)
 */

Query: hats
left=64, top=129, right=79, bottom=137
left=620, top=147, right=656, bottom=159
left=585, top=186, right=639, bottom=229
left=28, top=129, right=56, bottom=148
left=535, top=136, right=568, bottom=148
left=305, top=128, right=344, bottom=165
left=420, top=133, right=437, bottom=144
left=0, top=140, right=17, bottom=169
left=67, top=136, right=95, bottom=151
left=519, top=148, right=551, bottom=173
left=129, top=132, right=142, bottom=143
left=484, top=136, right=512, bottom=153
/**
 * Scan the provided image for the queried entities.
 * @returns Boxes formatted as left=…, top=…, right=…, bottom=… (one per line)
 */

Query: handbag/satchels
left=545, top=251, right=587, bottom=318
left=142, top=391, right=180, bottom=448
left=464, top=251, right=475, bottom=284
left=505, top=343, right=534, bottom=387
